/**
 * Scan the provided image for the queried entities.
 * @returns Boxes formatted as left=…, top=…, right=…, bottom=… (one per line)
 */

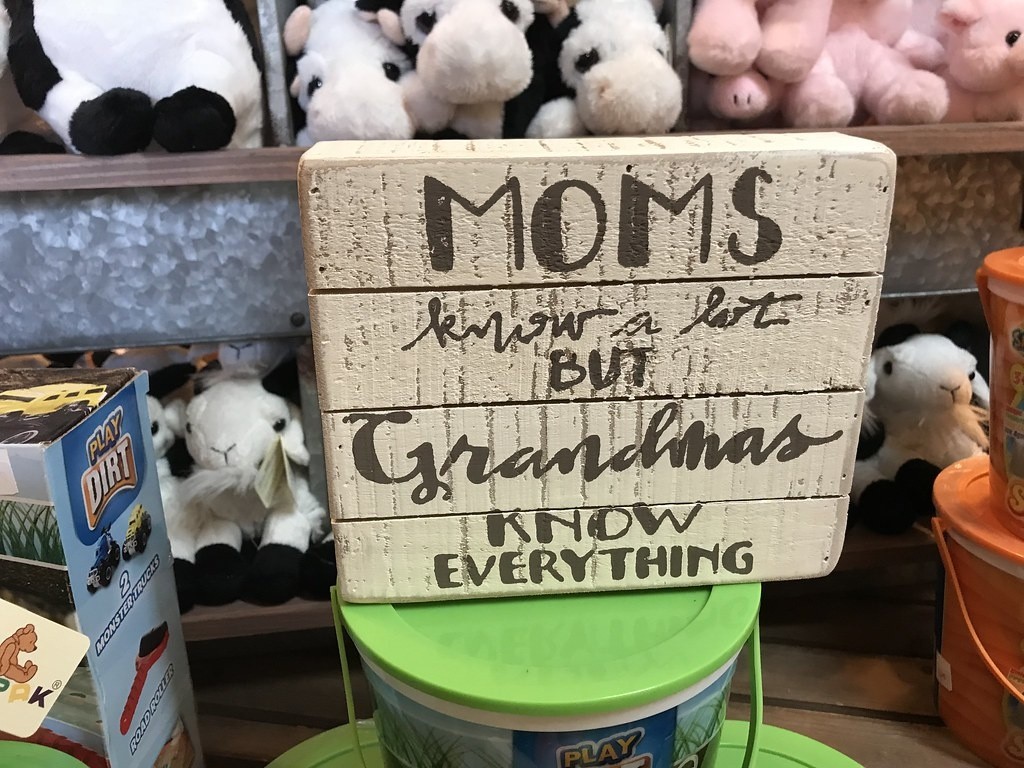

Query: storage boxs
left=0, top=365, right=202, bottom=768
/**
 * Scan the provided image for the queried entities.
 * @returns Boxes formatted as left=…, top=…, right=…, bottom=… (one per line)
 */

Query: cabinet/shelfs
left=0, top=1, right=1024, bottom=639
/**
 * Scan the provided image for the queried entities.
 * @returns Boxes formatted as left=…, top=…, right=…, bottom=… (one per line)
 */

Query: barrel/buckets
left=927, top=244, right=1024, bottom=768
left=263, top=563, right=869, bottom=768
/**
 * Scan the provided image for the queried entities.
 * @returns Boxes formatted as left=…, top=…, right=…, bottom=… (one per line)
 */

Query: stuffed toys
left=847, top=325, right=992, bottom=532
left=0, top=0, right=1023, bottom=156
left=0, top=336, right=340, bottom=614
